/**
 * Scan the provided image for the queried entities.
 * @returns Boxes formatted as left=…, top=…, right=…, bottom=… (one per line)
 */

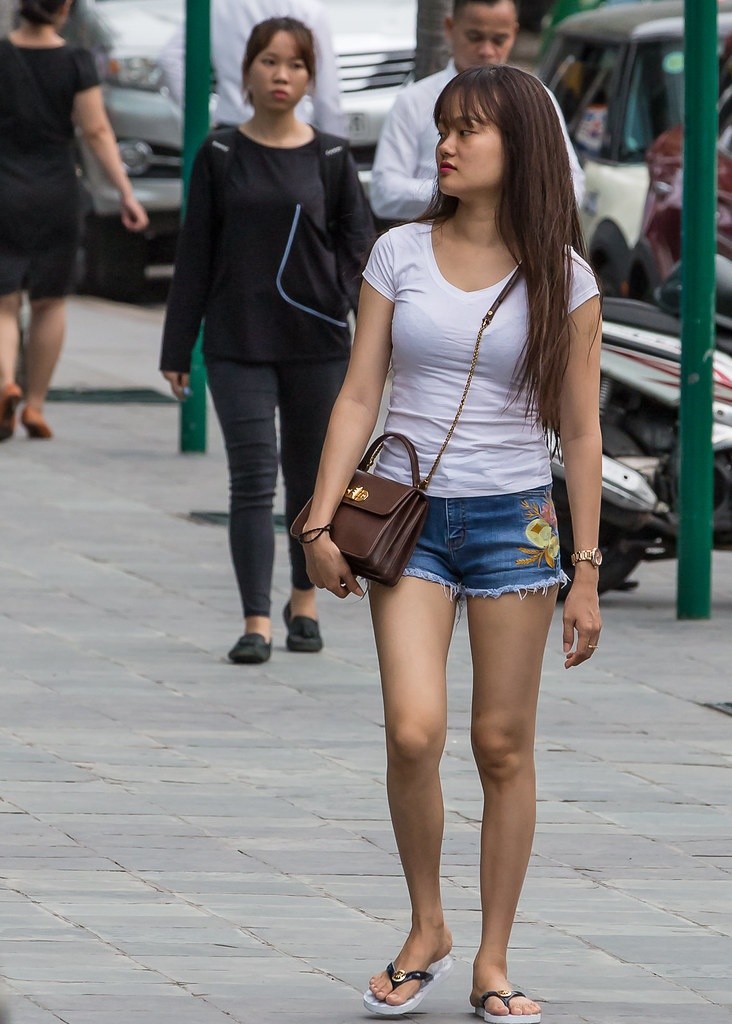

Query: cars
left=1, top=0, right=418, bottom=307
left=541, top=1, right=732, bottom=296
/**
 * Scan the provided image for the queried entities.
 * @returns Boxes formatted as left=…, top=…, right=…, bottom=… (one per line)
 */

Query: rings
left=588, top=644, right=599, bottom=651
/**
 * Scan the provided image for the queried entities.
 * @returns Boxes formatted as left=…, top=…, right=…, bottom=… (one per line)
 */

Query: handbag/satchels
left=290, top=433, right=430, bottom=588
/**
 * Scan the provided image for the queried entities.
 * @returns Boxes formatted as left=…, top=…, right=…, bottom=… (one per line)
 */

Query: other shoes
left=0, top=384, right=23, bottom=440
left=284, top=598, right=323, bottom=653
left=229, top=633, right=271, bottom=664
left=22, top=404, right=52, bottom=439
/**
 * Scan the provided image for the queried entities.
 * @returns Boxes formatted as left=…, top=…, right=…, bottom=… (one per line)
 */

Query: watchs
left=571, top=547, right=604, bottom=570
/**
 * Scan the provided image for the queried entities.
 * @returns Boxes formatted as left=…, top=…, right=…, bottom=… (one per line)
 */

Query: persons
left=159, top=0, right=377, bottom=662
left=0, top=1, right=150, bottom=439
left=370, top=0, right=588, bottom=223
left=302, top=64, right=606, bottom=1024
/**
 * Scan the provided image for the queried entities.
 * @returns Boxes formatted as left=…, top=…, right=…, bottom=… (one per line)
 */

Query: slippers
left=475, top=990, right=541, bottom=1023
left=363, top=954, right=453, bottom=1015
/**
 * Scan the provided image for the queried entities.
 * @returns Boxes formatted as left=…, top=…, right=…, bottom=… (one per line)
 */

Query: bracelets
left=299, top=523, right=332, bottom=544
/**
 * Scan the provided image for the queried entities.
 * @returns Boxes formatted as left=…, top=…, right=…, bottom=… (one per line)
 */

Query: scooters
left=548, top=295, right=732, bottom=605
